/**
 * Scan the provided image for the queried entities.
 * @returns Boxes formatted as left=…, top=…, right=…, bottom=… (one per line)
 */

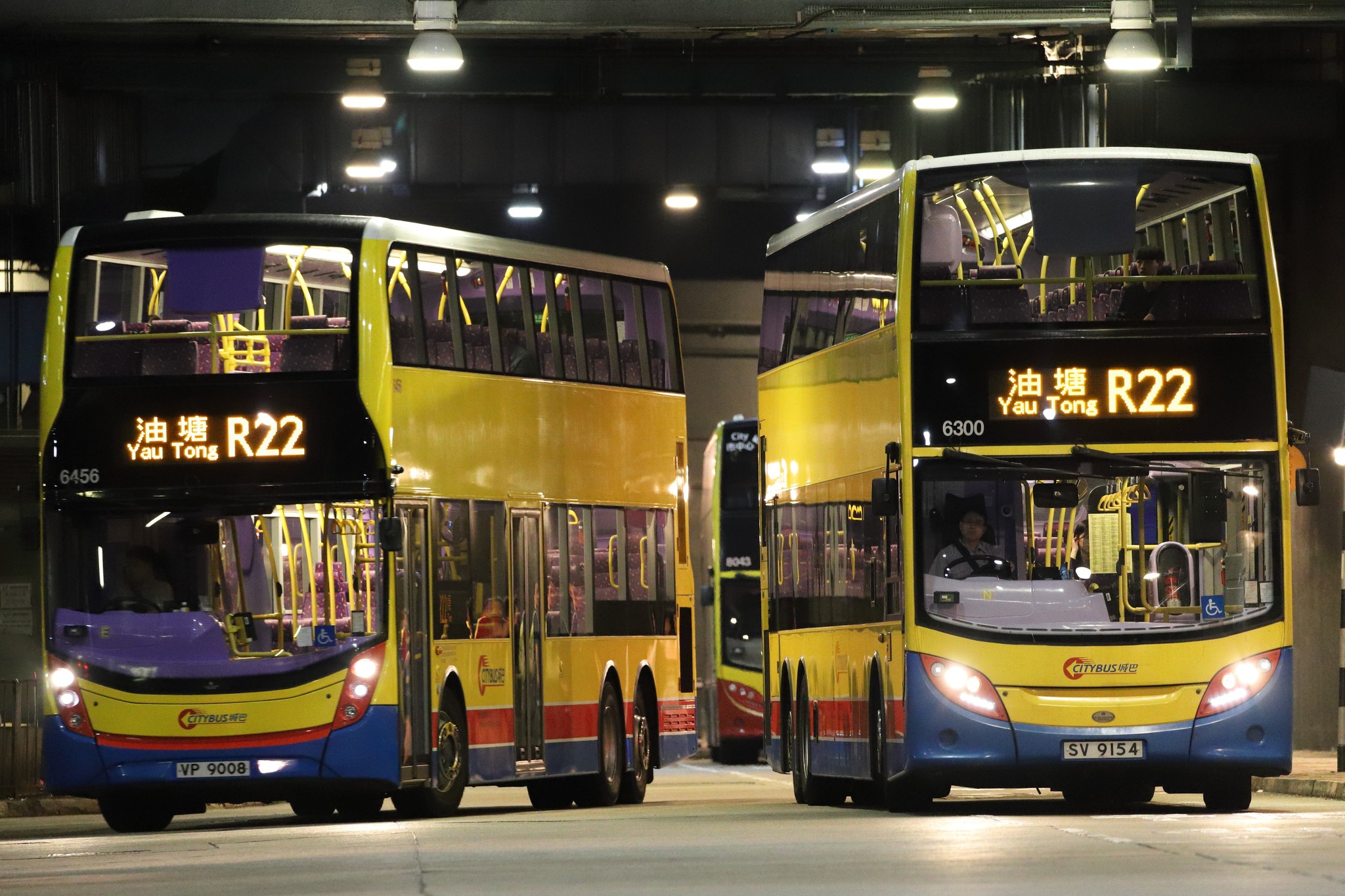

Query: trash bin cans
left=1, top=723, right=43, bottom=786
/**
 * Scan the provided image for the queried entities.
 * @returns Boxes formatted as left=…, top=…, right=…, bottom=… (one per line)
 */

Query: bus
left=757, top=145, right=1325, bottom=812
left=36, top=213, right=719, bottom=832
left=698, top=417, right=766, bottom=764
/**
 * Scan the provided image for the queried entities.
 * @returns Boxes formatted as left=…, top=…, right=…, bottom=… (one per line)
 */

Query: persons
left=513, top=579, right=544, bottom=754
left=479, top=596, right=524, bottom=638
left=546, top=582, right=562, bottom=636
left=928, top=505, right=1015, bottom=579
left=1114, top=244, right=1176, bottom=328
left=106, top=545, right=175, bottom=614
left=1070, top=520, right=1136, bottom=623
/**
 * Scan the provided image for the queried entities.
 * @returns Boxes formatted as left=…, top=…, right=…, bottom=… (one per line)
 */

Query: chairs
left=913, top=262, right=1256, bottom=326
left=301, top=544, right=665, bottom=638
left=74, top=314, right=670, bottom=396
left=780, top=526, right=1063, bottom=593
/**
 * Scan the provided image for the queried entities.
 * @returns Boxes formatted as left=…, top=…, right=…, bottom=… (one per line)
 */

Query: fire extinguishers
left=1165, top=565, right=1181, bottom=607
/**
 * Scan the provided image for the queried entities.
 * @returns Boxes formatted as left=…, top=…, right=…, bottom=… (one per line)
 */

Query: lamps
left=338, top=57, right=385, bottom=111
left=856, top=129, right=896, bottom=181
left=665, top=181, right=700, bottom=210
left=911, top=64, right=959, bottom=110
left=408, top=1, right=465, bottom=71
left=505, top=179, right=543, bottom=218
left=1106, top=0, right=1164, bottom=74
left=812, top=128, right=851, bottom=174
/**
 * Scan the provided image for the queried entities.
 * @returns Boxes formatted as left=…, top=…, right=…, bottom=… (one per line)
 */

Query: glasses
left=962, top=519, right=985, bottom=528
